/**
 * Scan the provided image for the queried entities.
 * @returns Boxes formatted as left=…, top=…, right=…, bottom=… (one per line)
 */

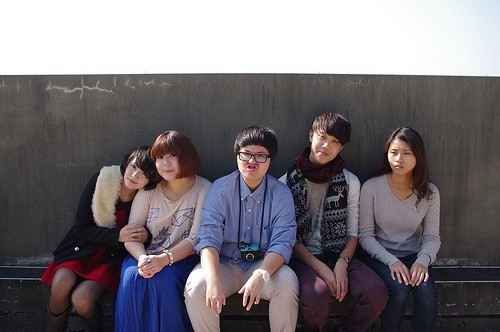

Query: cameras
left=240, top=243, right=260, bottom=261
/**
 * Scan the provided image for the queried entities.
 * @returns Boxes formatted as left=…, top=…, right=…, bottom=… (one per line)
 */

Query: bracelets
left=161, top=249, right=174, bottom=266
left=339, top=255, right=351, bottom=264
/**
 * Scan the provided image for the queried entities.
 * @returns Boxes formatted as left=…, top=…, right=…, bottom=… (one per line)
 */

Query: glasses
left=236, top=151, right=272, bottom=163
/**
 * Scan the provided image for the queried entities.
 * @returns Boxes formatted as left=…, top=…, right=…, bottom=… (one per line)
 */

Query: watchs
left=254, top=268, right=271, bottom=284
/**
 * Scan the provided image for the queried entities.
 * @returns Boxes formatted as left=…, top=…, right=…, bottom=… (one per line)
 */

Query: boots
left=46, top=299, right=73, bottom=332
left=80, top=302, right=104, bottom=332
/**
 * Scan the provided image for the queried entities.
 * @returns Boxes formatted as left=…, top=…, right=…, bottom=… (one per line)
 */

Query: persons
left=355, top=128, right=442, bottom=332
left=45, top=147, right=160, bottom=332
left=184, top=127, right=300, bottom=332
left=116, top=130, right=213, bottom=332
left=274, top=111, right=388, bottom=332
